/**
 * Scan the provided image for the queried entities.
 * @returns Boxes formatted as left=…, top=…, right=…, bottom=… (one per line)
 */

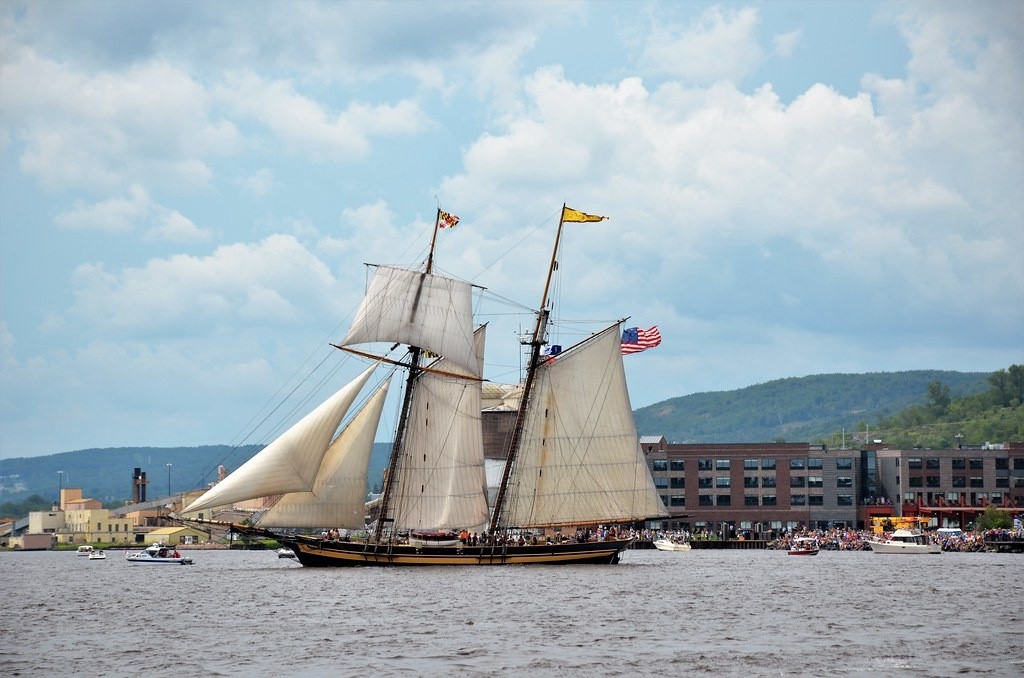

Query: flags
left=544, top=344, right=562, bottom=356
left=620, top=325, right=662, bottom=355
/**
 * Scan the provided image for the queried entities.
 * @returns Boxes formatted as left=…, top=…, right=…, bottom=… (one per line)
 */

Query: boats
left=653, top=540, right=691, bottom=551
left=126, top=543, right=195, bottom=567
left=279, top=549, right=295, bottom=558
left=78, top=546, right=94, bottom=555
left=89, top=551, right=105, bottom=559
left=868, top=541, right=941, bottom=554
left=787, top=538, right=820, bottom=555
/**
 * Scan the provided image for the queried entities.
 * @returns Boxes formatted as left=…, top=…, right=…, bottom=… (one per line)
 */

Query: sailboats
left=156, top=203, right=696, bottom=566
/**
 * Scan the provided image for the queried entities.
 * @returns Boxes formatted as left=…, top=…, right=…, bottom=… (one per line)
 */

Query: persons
left=162, top=550, right=180, bottom=558
left=766, top=525, right=894, bottom=551
left=455, top=524, right=724, bottom=547
left=918, top=516, right=1024, bottom=552
left=321, top=528, right=351, bottom=542
left=864, top=496, right=893, bottom=506
